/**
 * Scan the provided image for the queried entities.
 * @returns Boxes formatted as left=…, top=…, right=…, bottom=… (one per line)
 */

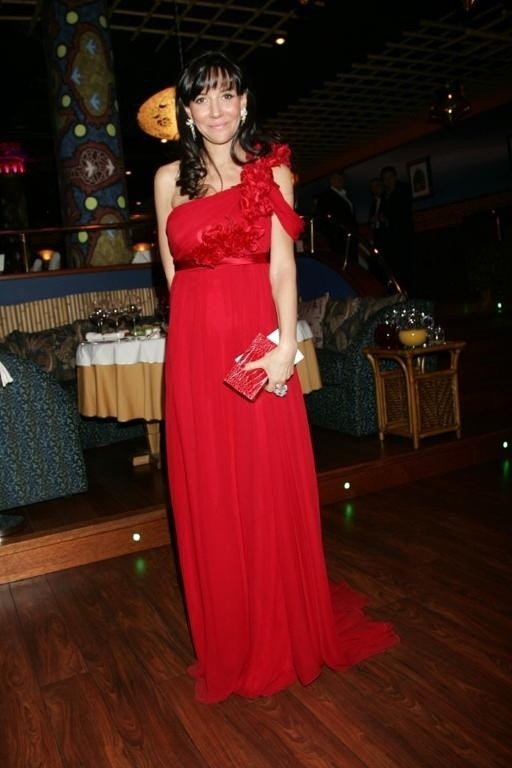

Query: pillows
left=301, top=292, right=407, bottom=354
left=5, top=319, right=101, bottom=387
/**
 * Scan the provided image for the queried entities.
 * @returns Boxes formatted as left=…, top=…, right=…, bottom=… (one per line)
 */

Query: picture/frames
left=406, top=156, right=431, bottom=200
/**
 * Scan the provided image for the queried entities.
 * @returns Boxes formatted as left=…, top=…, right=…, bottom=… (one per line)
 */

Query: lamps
left=137, top=0, right=188, bottom=142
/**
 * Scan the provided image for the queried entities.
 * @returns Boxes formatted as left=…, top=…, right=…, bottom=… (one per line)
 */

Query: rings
left=274, top=384, right=288, bottom=396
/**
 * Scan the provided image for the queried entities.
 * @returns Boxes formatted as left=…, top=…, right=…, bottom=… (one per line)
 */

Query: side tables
left=364, top=340, right=467, bottom=449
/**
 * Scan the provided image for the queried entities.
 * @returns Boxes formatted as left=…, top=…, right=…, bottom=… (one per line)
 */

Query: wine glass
left=85, top=302, right=150, bottom=344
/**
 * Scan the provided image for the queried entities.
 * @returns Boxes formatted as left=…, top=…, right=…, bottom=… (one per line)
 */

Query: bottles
left=373, top=308, right=436, bottom=349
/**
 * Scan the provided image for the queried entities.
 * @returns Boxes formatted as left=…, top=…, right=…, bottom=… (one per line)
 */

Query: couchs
left=1, top=350, right=146, bottom=508
left=301, top=296, right=433, bottom=440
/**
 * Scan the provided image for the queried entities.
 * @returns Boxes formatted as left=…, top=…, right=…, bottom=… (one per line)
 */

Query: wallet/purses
left=225, top=332, right=279, bottom=402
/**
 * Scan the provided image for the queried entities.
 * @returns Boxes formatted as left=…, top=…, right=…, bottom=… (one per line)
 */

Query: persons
left=151, top=47, right=400, bottom=701
left=316, top=157, right=416, bottom=295
